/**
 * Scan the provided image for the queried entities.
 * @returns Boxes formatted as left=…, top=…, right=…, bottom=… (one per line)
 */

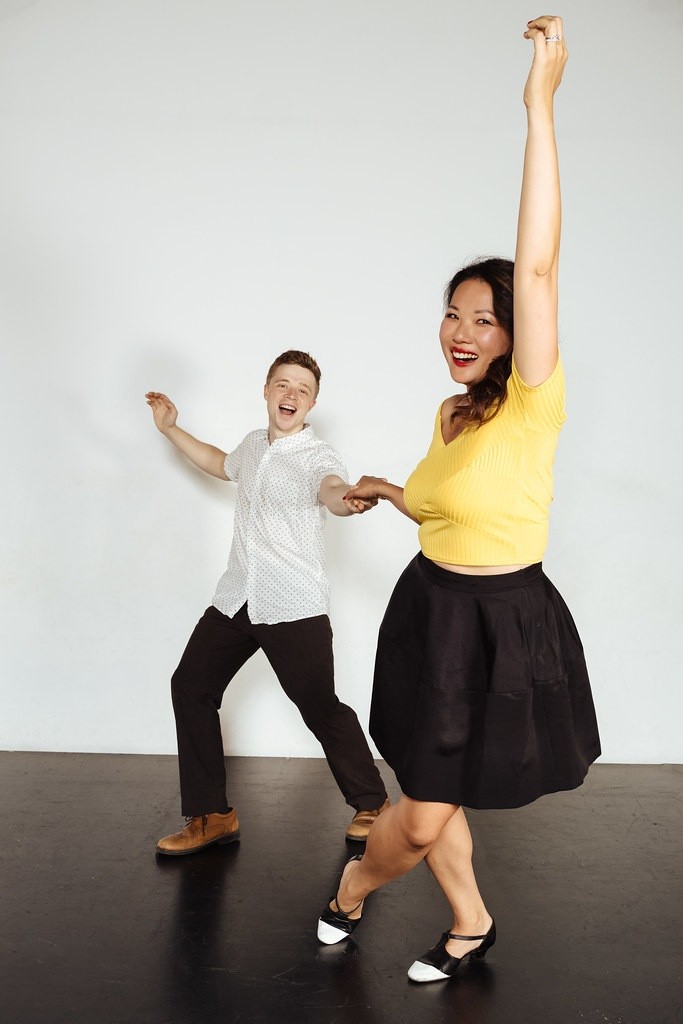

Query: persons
left=145, top=350, right=389, bottom=856
left=319, top=12, right=604, bottom=985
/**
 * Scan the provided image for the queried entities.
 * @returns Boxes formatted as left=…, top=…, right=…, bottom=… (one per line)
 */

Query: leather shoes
left=156, top=807, right=238, bottom=855
left=346, top=797, right=391, bottom=842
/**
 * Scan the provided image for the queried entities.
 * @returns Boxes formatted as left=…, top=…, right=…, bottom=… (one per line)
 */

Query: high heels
left=317, top=854, right=365, bottom=945
left=407, top=915, right=496, bottom=982
